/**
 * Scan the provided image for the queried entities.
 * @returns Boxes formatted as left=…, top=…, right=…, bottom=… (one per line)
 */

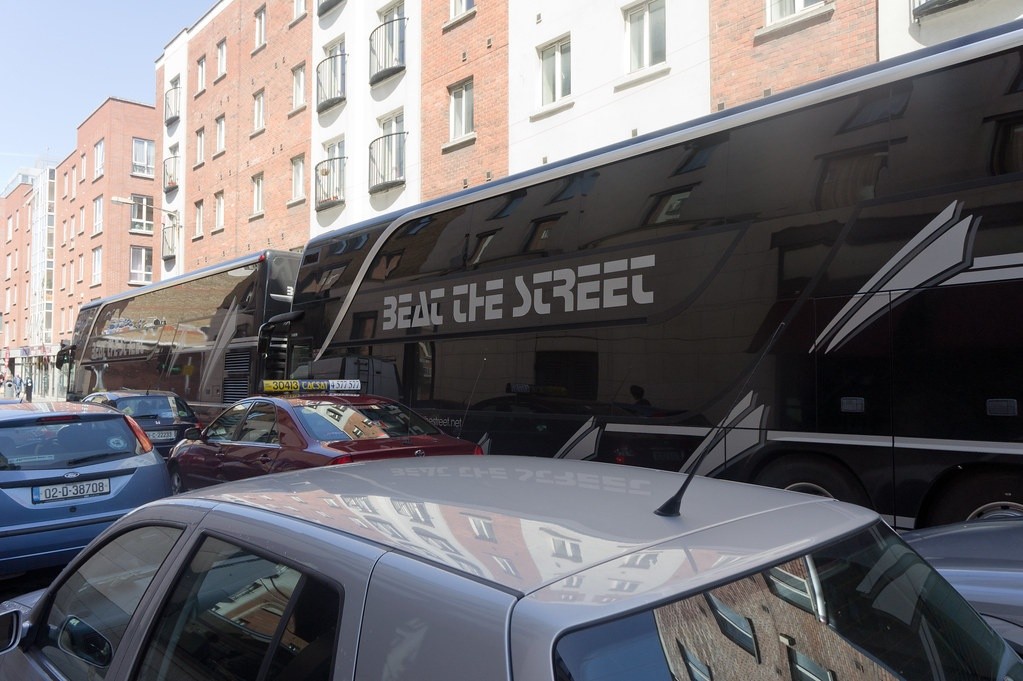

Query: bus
left=255, top=19, right=1023, bottom=534
left=56, top=251, right=303, bottom=431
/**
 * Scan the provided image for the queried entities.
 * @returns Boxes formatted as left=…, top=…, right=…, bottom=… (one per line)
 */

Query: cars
left=0, top=398, right=169, bottom=581
left=896, top=521, right=1023, bottom=658
left=0, top=455, right=1023, bottom=681
left=167, top=381, right=484, bottom=496
left=57, top=390, right=205, bottom=463
